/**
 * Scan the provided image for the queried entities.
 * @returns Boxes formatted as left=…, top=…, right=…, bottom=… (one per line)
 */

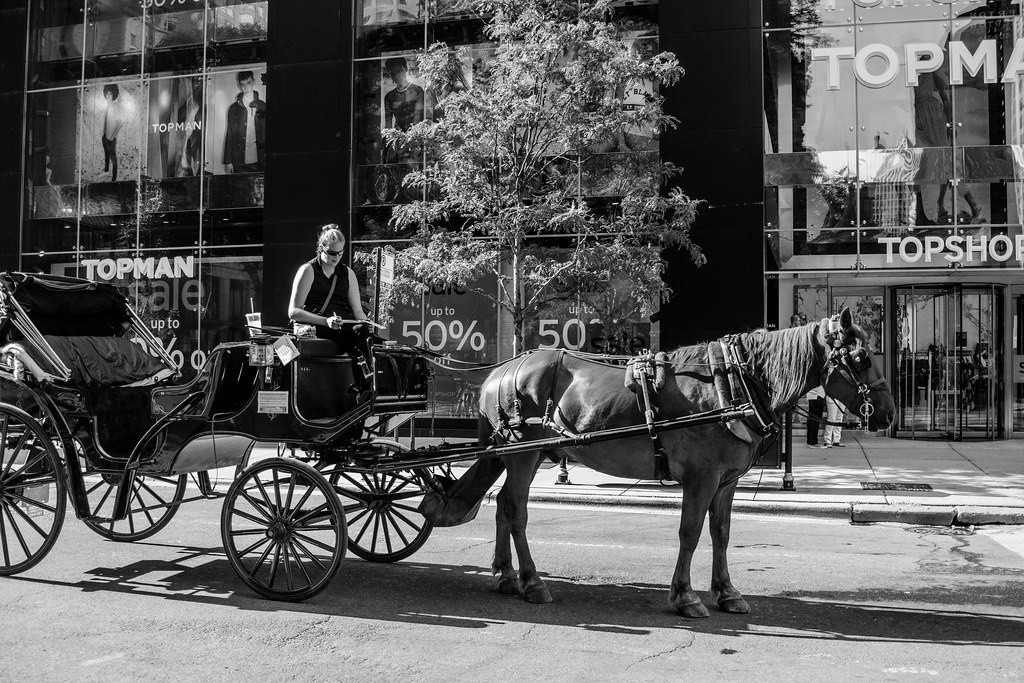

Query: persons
left=383, top=54, right=473, bottom=169
left=977, top=348, right=997, bottom=403
left=223, top=70, right=266, bottom=174
left=37, top=154, right=60, bottom=218
left=804, top=383, right=846, bottom=449
left=287, top=223, right=371, bottom=407
left=99, top=85, right=122, bottom=181
left=915, top=56, right=981, bottom=225
left=163, top=77, right=201, bottom=178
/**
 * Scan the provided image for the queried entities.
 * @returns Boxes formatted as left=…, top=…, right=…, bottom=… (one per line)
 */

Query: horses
left=478, top=306, right=896, bottom=617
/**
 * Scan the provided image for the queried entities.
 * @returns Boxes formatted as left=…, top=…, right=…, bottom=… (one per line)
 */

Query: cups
left=245, top=312, right=262, bottom=336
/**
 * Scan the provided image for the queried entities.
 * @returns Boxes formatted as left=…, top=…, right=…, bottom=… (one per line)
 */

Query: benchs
left=245, top=324, right=345, bottom=357
left=151, top=340, right=258, bottom=413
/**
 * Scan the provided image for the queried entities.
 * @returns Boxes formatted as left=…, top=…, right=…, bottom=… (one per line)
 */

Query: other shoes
left=807, top=444, right=816, bottom=449
left=816, top=444, right=827, bottom=449
left=832, top=442, right=845, bottom=448
left=823, top=443, right=833, bottom=448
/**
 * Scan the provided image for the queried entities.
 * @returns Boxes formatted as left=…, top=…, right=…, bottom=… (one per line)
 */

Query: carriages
left=0, top=270, right=897, bottom=618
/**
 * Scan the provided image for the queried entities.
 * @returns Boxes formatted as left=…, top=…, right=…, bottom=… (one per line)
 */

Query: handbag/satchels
left=294, top=322, right=317, bottom=339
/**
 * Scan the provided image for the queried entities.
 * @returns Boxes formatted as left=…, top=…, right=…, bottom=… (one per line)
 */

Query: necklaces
left=396, top=82, right=411, bottom=91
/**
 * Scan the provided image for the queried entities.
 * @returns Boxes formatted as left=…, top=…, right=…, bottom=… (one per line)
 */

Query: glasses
left=324, top=249, right=345, bottom=255
